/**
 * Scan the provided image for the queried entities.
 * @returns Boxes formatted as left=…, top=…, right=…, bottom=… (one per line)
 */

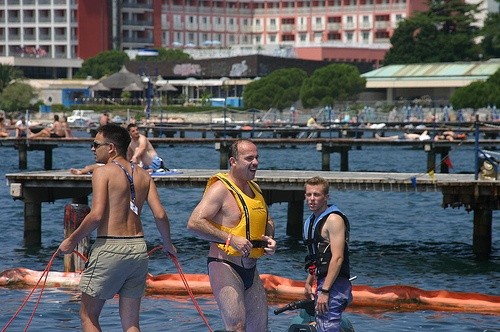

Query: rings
left=245, top=250, right=247, bottom=252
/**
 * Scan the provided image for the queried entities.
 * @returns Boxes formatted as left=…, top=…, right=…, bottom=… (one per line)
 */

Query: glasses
left=91, top=142, right=116, bottom=149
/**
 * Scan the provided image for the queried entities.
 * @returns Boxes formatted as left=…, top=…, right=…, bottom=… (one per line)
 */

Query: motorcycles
left=273, top=275, right=359, bottom=332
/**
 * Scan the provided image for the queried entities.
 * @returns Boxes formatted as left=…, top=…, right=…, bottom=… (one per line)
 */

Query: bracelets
left=321, top=289, right=329, bottom=293
left=226, top=234, right=232, bottom=246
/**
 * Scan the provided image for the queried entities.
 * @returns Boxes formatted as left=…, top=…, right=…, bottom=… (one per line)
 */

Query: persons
left=436, top=131, right=454, bottom=141
left=187, top=138, right=278, bottom=332
left=303, top=176, right=352, bottom=332
left=101, top=114, right=109, bottom=125
left=127, top=124, right=161, bottom=173
left=375, top=131, right=428, bottom=139
left=30, top=115, right=71, bottom=138
left=71, top=164, right=105, bottom=175
left=308, top=118, right=317, bottom=127
left=16, top=117, right=32, bottom=137
left=443, top=105, right=450, bottom=122
left=0, top=111, right=10, bottom=137
left=60, top=124, right=177, bottom=332
